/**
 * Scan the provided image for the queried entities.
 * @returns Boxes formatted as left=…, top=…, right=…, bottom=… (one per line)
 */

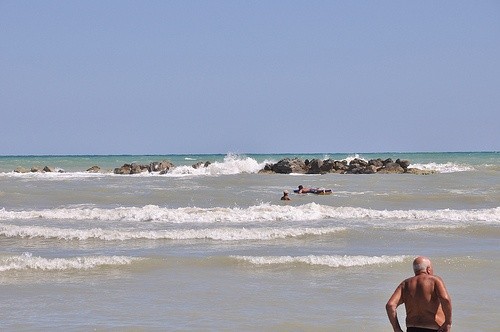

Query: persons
left=386, top=256, right=453, bottom=331
left=281, top=191, right=290, bottom=200
left=298, top=184, right=326, bottom=193
